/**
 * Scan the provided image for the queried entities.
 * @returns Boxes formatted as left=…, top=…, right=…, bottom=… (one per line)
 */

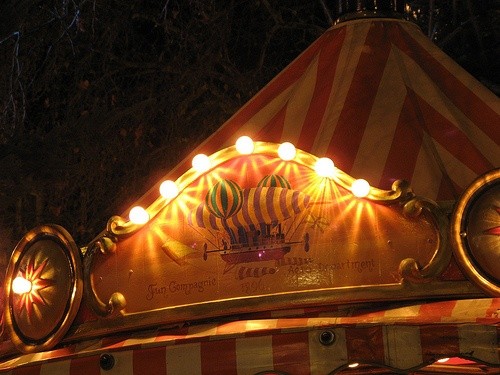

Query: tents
left=1, top=16, right=500, bottom=375
